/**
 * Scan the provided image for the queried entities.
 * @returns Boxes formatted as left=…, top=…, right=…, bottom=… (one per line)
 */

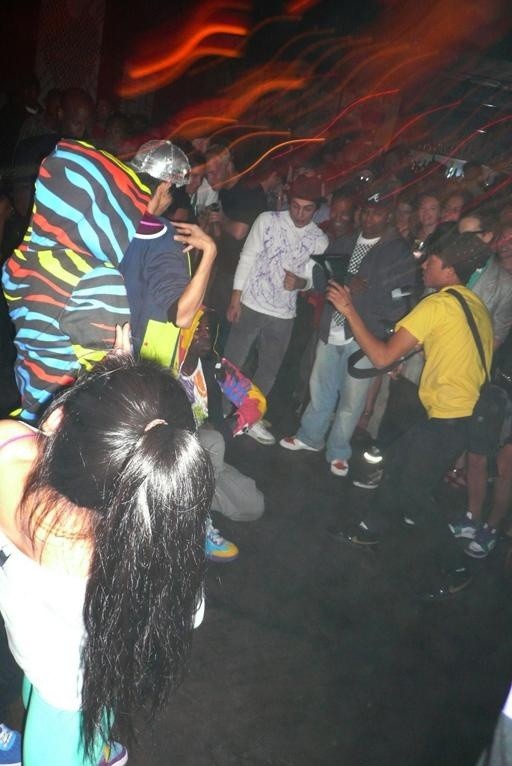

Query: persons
left=177, top=307, right=268, bottom=561
left=280, top=190, right=422, bottom=476
left=0, top=136, right=151, bottom=424
left=407, top=193, right=443, bottom=262
left=0, top=356, right=217, bottom=765
left=447, top=225, right=512, bottom=562
left=395, top=192, right=419, bottom=237
left=316, top=186, right=362, bottom=242
left=354, top=208, right=512, bottom=489
left=173, top=137, right=219, bottom=218
left=120, top=140, right=217, bottom=430
left=324, top=234, right=495, bottom=596
left=266, top=141, right=512, bottom=196
left=220, top=175, right=329, bottom=442
left=206, top=146, right=265, bottom=357
left=442, top=191, right=474, bottom=223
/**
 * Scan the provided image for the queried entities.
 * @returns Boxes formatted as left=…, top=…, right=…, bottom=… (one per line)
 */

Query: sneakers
left=403, top=514, right=416, bottom=529
left=353, top=468, right=384, bottom=489
left=440, top=569, right=475, bottom=593
left=465, top=523, right=499, bottom=558
left=331, top=459, right=349, bottom=477
left=0, top=723, right=22, bottom=765
left=279, top=435, right=320, bottom=452
left=363, top=446, right=383, bottom=464
left=243, top=421, right=275, bottom=445
left=98, top=741, right=128, bottom=766
left=205, top=526, right=239, bottom=563
left=449, top=520, right=478, bottom=543
left=192, top=587, right=205, bottom=629
left=340, top=527, right=384, bottom=545
left=263, top=416, right=273, bottom=428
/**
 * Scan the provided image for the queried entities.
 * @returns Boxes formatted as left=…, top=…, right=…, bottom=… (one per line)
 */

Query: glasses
left=472, top=230, right=485, bottom=235
left=360, top=176, right=371, bottom=182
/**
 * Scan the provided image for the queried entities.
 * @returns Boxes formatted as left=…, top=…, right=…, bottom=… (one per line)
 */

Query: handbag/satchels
left=445, top=289, right=512, bottom=456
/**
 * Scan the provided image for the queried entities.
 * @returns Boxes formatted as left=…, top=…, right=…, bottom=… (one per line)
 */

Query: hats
left=284, top=174, right=328, bottom=204
left=124, top=139, right=192, bottom=188
left=365, top=189, right=394, bottom=210
left=134, top=211, right=168, bottom=239
left=429, top=231, right=492, bottom=270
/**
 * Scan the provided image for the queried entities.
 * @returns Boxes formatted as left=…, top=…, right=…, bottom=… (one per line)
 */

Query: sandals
left=452, top=471, right=467, bottom=490
left=360, top=412, right=371, bottom=430
left=442, top=468, right=463, bottom=484
